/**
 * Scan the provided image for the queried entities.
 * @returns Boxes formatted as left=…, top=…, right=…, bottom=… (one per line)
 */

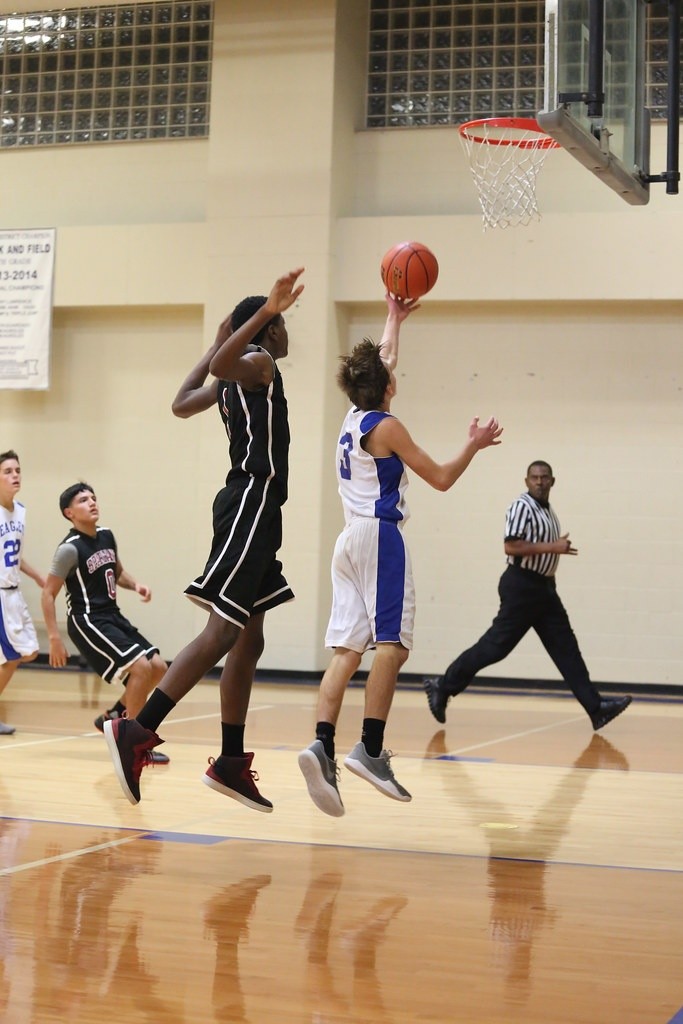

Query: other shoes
left=0, top=722, right=15, bottom=734
left=94, top=710, right=116, bottom=733
left=143, top=751, right=169, bottom=764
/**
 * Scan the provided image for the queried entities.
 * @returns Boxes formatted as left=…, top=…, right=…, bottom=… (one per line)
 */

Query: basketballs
left=380, top=239, right=439, bottom=299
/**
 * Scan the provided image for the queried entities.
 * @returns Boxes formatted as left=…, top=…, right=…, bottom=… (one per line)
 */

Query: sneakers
left=297, top=740, right=344, bottom=818
left=201, top=752, right=273, bottom=813
left=103, top=718, right=166, bottom=804
left=343, top=742, right=412, bottom=803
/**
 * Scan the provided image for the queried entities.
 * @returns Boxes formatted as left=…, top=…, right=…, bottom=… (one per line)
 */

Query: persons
left=103, top=266, right=305, bottom=813
left=422, top=460, right=634, bottom=730
left=0, top=450, right=46, bottom=735
left=296, top=287, right=504, bottom=818
left=40, top=481, right=170, bottom=766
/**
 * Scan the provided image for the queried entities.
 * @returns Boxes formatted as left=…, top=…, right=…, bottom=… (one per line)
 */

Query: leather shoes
left=423, top=678, right=450, bottom=724
left=588, top=695, right=632, bottom=730
